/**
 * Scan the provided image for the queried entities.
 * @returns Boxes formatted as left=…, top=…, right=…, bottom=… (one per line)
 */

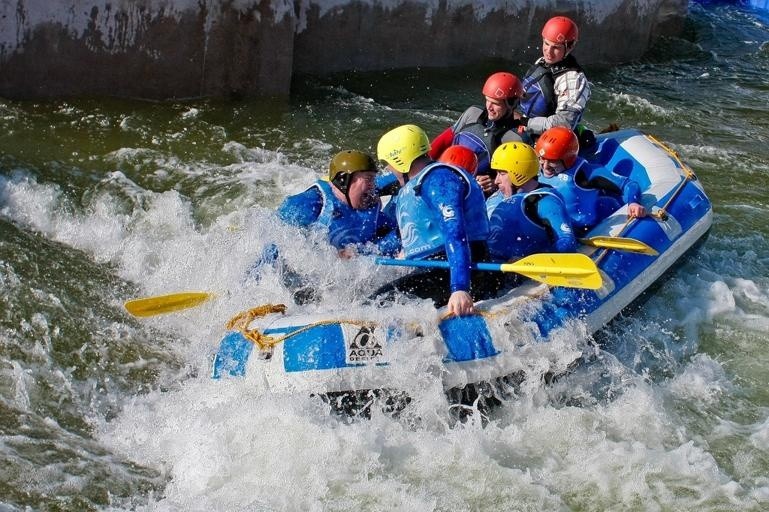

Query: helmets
left=437, top=146, right=479, bottom=178
left=328, top=150, right=379, bottom=181
left=541, top=16, right=579, bottom=43
left=376, top=125, right=432, bottom=175
left=481, top=72, right=524, bottom=99
left=490, top=141, right=540, bottom=187
left=534, top=126, right=580, bottom=170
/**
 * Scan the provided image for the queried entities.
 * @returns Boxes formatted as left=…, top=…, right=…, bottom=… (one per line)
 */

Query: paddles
left=123, top=292, right=216, bottom=318
left=578, top=235, right=658, bottom=258
left=373, top=252, right=604, bottom=290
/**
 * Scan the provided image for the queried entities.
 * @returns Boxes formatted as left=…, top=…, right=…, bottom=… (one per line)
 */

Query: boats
left=216, top=129, right=716, bottom=425
